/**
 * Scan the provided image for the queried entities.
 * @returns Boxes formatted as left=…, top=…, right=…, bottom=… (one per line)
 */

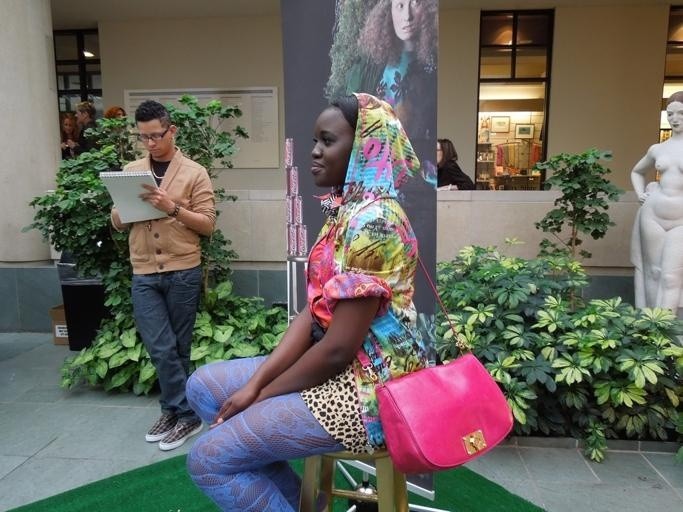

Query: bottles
left=479, top=119, right=489, bottom=142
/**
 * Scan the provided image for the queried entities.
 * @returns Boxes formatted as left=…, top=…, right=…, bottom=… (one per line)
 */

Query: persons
left=630, top=91, right=683, bottom=316
left=104, top=106, right=126, bottom=119
left=324, top=0, right=438, bottom=214
left=436, top=139, right=475, bottom=191
left=74, top=101, right=101, bottom=154
left=59, top=111, right=80, bottom=160
left=110, top=101, right=217, bottom=451
left=184, top=92, right=430, bottom=512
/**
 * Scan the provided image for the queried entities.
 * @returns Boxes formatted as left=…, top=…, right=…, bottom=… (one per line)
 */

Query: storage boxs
left=50, top=304, right=69, bottom=345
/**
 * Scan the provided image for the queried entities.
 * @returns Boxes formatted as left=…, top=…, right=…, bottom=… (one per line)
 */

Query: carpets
left=0, top=453, right=551, bottom=512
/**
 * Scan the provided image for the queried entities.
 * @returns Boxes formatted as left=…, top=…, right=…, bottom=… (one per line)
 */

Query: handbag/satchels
left=375, top=353, right=514, bottom=475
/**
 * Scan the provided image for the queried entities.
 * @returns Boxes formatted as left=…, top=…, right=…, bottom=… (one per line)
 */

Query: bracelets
left=167, top=203, right=180, bottom=217
left=448, top=184, right=452, bottom=191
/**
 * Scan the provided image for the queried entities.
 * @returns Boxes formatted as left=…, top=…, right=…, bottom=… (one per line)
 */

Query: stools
left=300, top=447, right=408, bottom=512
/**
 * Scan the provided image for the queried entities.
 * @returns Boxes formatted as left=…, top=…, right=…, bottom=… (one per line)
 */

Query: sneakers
left=145, top=414, right=177, bottom=442
left=159, top=416, right=207, bottom=450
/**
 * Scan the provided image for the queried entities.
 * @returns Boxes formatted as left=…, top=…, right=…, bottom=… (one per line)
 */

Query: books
left=98, top=170, right=170, bottom=224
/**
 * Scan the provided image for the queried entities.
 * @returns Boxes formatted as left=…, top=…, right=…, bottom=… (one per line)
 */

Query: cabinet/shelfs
left=476, top=142, right=495, bottom=183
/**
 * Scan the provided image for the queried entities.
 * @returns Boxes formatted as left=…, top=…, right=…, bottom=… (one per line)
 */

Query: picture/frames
left=514, top=123, right=535, bottom=139
left=491, top=116, right=510, bottom=133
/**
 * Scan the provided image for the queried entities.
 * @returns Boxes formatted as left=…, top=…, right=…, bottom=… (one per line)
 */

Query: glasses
left=137, top=126, right=169, bottom=142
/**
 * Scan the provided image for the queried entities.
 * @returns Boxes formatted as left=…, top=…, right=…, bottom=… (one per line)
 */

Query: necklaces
left=151, top=163, right=164, bottom=179
